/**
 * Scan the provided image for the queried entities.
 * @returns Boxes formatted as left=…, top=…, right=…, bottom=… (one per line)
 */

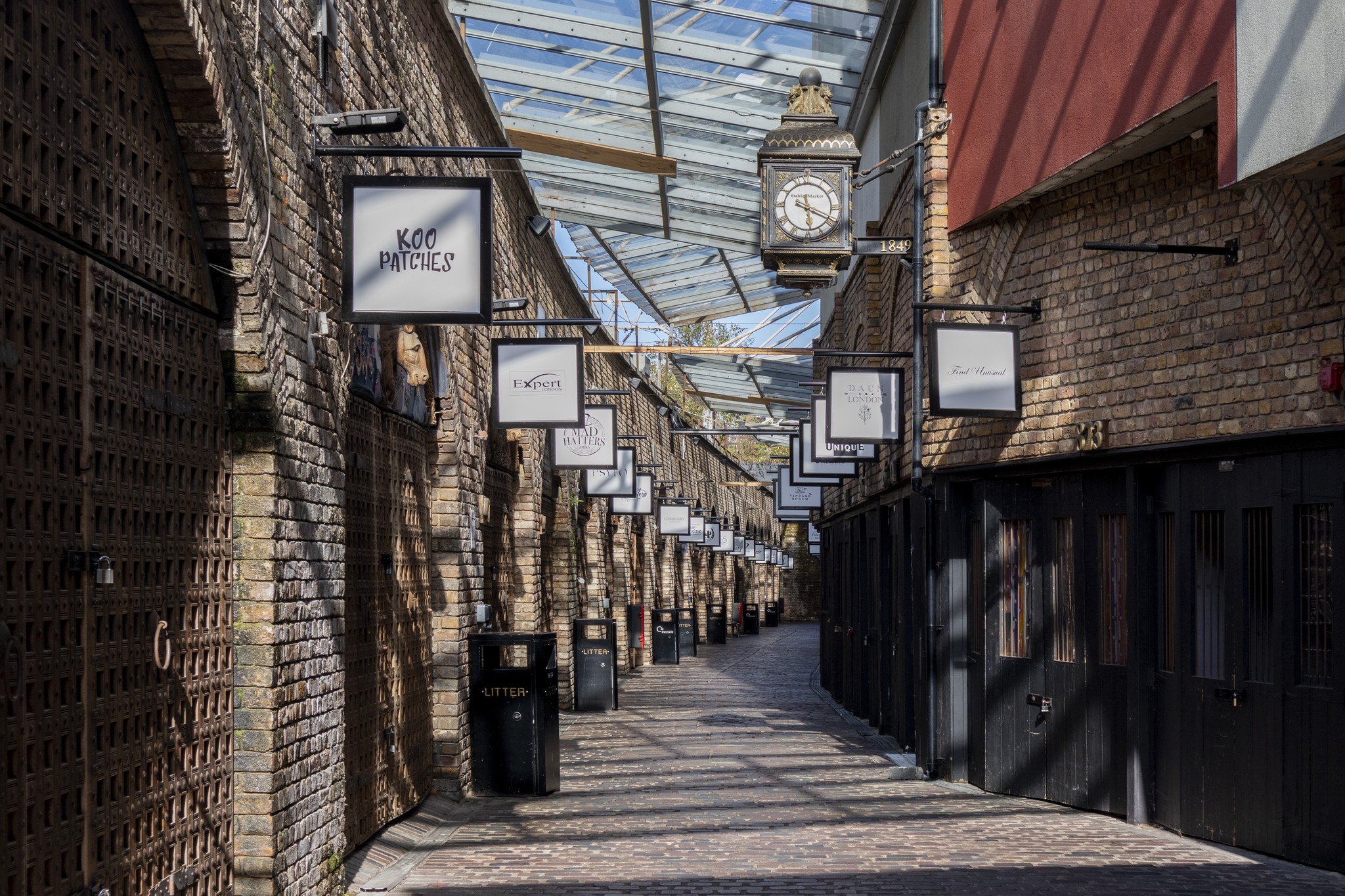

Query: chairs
left=726, top=617, right=741, bottom=640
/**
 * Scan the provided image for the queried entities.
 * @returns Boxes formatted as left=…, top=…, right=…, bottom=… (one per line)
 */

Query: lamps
left=582, top=325, right=601, bottom=336
left=762, top=491, right=767, bottom=496
left=628, top=377, right=642, bottom=389
left=526, top=215, right=552, bottom=240
left=690, top=508, right=706, bottom=510
left=755, top=486, right=762, bottom=491
left=698, top=473, right=714, bottom=483
left=493, top=298, right=528, bottom=312
left=654, top=486, right=674, bottom=490
left=725, top=514, right=730, bottom=519
left=720, top=459, right=727, bottom=465
left=672, top=499, right=688, bottom=502
left=692, top=437, right=701, bottom=445
left=310, top=108, right=410, bottom=134
left=748, top=507, right=758, bottom=509
left=657, top=405, right=668, bottom=416
left=735, top=470, right=742, bottom=476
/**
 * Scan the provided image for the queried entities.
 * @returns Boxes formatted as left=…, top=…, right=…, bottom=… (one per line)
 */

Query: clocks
left=774, top=168, right=842, bottom=243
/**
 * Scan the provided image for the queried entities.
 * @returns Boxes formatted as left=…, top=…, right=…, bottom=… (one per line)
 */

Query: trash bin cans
left=673, top=607, right=696, bottom=657
left=743, top=603, right=759, bottom=634
left=764, top=601, right=778, bottom=626
left=651, top=609, right=679, bottom=665
left=573, top=618, right=617, bottom=711
left=467, top=632, right=562, bottom=797
left=707, top=603, right=727, bottom=644
